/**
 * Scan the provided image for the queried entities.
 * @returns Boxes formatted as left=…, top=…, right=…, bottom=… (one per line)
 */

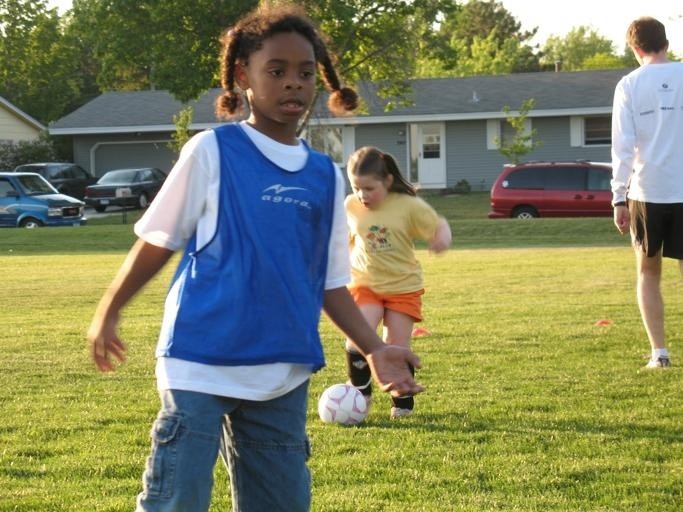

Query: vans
left=488, top=159, right=613, bottom=220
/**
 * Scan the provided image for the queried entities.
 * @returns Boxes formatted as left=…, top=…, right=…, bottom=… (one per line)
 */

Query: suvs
left=0, top=173, right=85, bottom=230
left=15, top=162, right=100, bottom=201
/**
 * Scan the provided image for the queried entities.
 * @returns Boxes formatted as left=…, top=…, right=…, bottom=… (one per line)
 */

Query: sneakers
left=645, top=355, right=669, bottom=367
left=390, top=407, right=413, bottom=418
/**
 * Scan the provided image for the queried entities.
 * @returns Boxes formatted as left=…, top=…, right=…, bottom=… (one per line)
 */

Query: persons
left=83, top=1, right=425, bottom=511
left=342, top=146, right=453, bottom=421
left=609, top=17, right=682, bottom=371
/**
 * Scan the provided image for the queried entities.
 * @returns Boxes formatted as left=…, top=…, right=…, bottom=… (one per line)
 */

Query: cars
left=84, top=166, right=167, bottom=213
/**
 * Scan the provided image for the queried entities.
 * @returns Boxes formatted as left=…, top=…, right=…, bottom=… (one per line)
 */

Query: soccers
left=318, top=384, right=366, bottom=426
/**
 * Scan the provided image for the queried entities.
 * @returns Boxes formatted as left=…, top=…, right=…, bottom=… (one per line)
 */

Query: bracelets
left=612, top=200, right=626, bottom=207
